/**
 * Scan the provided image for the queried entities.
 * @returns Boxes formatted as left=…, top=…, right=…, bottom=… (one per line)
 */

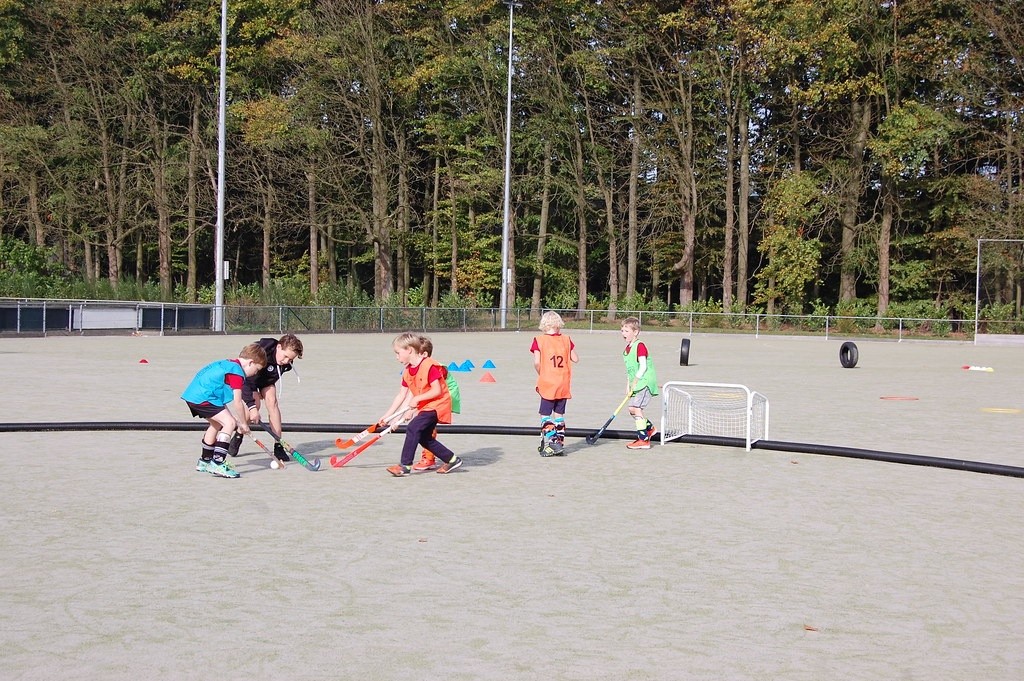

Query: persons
left=622, top=317, right=658, bottom=449
left=181, top=343, right=267, bottom=477
left=379, top=332, right=463, bottom=476
left=227, top=334, right=303, bottom=460
left=530, top=311, right=579, bottom=456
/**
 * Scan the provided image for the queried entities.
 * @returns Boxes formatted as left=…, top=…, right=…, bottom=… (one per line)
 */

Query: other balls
left=270, top=460, right=279, bottom=470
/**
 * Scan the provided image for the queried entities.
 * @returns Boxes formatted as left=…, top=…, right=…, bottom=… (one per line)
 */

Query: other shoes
left=413, top=460, right=436, bottom=470
left=227, top=433, right=242, bottom=457
left=273, top=450, right=290, bottom=461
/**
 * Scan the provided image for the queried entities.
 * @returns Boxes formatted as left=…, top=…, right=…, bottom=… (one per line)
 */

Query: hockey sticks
left=247, top=433, right=287, bottom=470
left=336, top=406, right=410, bottom=449
left=257, top=420, right=321, bottom=471
left=586, top=391, right=635, bottom=444
left=330, top=407, right=417, bottom=467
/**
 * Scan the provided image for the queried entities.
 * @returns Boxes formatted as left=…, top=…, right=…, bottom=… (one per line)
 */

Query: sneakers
left=626, top=438, right=651, bottom=449
left=205, top=459, right=240, bottom=478
left=386, top=464, right=410, bottom=476
left=195, top=456, right=213, bottom=472
left=647, top=426, right=657, bottom=440
left=436, top=456, right=463, bottom=473
left=540, top=441, right=563, bottom=457
